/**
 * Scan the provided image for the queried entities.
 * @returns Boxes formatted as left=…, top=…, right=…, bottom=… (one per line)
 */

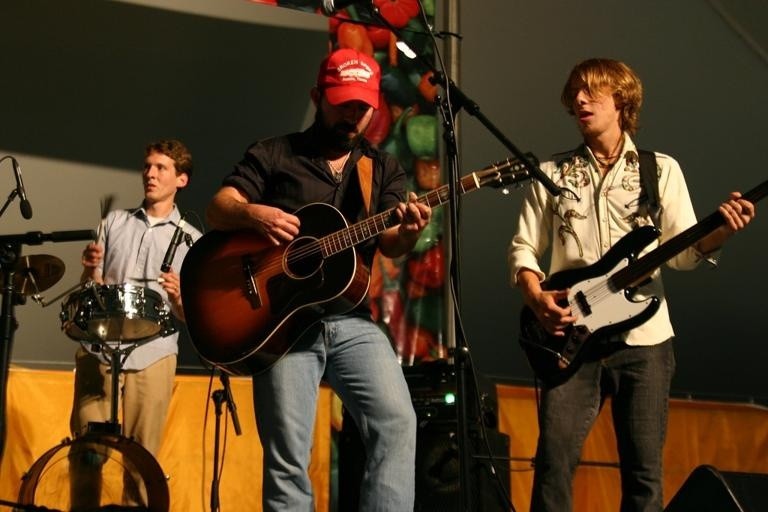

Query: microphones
left=321, top=0, right=368, bottom=17
left=160, top=211, right=188, bottom=273
left=12, top=159, right=32, bottom=219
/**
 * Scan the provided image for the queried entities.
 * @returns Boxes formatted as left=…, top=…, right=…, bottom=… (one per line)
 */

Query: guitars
left=180, top=151, right=540, bottom=377
left=517, top=182, right=766, bottom=386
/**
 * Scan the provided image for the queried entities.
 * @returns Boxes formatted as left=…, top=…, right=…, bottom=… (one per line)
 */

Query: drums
left=60, top=281, right=168, bottom=342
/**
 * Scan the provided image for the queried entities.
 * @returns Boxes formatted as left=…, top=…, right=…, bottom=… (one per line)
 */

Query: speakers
left=663, top=464, right=768, bottom=512
left=338, top=422, right=511, bottom=512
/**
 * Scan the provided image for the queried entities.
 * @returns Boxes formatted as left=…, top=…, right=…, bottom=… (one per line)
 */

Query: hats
left=318, top=48, right=380, bottom=108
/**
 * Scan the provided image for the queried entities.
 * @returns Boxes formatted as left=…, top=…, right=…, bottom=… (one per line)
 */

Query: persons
left=202, top=49, right=433, bottom=512
left=507, top=58, right=755, bottom=512
left=67, top=139, right=211, bottom=512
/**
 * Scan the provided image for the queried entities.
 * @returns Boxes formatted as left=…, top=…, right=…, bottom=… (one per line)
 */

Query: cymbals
left=1, top=255, right=64, bottom=296
